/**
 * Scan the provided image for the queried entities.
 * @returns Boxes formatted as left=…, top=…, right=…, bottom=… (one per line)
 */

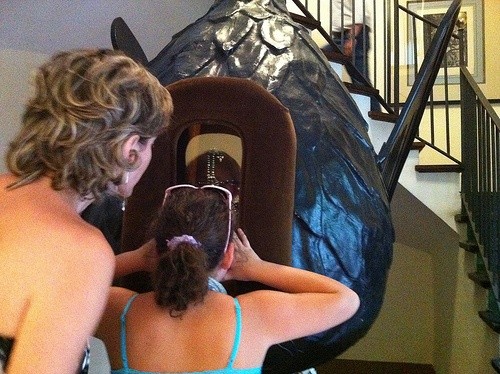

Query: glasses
left=162, top=184, right=233, bottom=253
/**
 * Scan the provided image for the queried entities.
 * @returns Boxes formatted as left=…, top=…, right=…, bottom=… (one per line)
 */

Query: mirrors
left=175, top=118, right=244, bottom=233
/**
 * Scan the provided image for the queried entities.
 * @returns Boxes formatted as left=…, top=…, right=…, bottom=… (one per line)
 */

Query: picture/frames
left=407, top=0, right=485, bottom=86
